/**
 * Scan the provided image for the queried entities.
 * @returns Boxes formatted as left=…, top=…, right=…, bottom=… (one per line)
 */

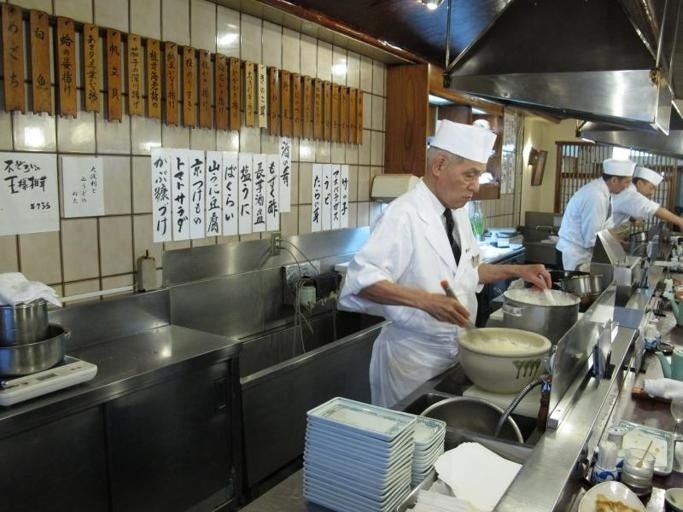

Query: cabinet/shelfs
left=4, top=387, right=110, bottom=511
left=478, top=243, right=526, bottom=308
left=102, top=343, right=248, bottom=511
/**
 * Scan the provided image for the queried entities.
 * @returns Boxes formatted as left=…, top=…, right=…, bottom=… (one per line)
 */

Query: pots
left=1, top=299, right=48, bottom=344
left=0, top=323, right=72, bottom=375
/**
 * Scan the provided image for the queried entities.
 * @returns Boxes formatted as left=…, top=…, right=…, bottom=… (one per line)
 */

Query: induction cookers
left=0, top=353, right=98, bottom=405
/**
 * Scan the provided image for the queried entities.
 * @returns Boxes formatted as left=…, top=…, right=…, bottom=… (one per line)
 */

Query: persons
left=595, top=166, right=682, bottom=259
left=336, top=119, right=551, bottom=409
left=555, top=157, right=635, bottom=280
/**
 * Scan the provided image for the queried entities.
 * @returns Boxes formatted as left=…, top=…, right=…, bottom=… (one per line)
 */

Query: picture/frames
left=532, top=150, right=547, bottom=186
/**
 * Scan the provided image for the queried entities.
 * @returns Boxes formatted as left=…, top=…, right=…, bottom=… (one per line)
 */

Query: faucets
left=536, top=225, right=553, bottom=233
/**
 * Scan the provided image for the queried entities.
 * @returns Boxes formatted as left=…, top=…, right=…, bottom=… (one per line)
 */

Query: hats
left=633, top=167, right=664, bottom=186
left=602, top=159, right=636, bottom=177
left=429, top=119, right=497, bottom=164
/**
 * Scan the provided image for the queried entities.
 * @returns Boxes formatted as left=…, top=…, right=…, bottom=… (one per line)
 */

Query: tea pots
left=655, top=346, right=683, bottom=380
left=669, top=297, right=682, bottom=324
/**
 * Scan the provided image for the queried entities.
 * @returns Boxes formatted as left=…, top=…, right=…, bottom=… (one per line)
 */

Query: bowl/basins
left=630, top=231, right=648, bottom=242
left=559, top=275, right=603, bottom=306
left=457, top=328, right=552, bottom=393
left=420, top=397, right=524, bottom=447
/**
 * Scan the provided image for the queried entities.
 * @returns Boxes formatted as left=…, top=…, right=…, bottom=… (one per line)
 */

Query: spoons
left=635, top=440, right=653, bottom=468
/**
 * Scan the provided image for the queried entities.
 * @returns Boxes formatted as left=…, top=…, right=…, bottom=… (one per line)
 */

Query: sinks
left=432, top=367, right=474, bottom=396
left=526, top=234, right=548, bottom=243
left=393, top=392, right=540, bottom=449
left=579, top=280, right=633, bottom=313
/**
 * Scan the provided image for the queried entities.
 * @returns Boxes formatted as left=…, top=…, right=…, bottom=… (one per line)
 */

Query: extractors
left=440, top=0, right=678, bottom=137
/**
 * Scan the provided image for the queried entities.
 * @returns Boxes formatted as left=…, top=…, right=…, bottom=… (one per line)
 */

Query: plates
left=579, top=482, right=648, bottom=512
left=303, top=397, right=417, bottom=512
left=603, top=421, right=674, bottom=474
left=411, top=416, right=447, bottom=486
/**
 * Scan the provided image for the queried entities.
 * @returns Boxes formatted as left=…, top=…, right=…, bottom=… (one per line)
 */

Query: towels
left=623, top=428, right=667, bottom=470
left=0, top=272, right=62, bottom=308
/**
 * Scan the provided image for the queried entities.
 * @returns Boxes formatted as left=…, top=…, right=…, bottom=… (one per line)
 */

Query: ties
left=606, top=195, right=612, bottom=221
left=443, top=208, right=462, bottom=267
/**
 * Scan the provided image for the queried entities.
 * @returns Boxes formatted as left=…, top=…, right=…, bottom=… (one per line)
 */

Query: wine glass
left=670, top=392, right=683, bottom=439
